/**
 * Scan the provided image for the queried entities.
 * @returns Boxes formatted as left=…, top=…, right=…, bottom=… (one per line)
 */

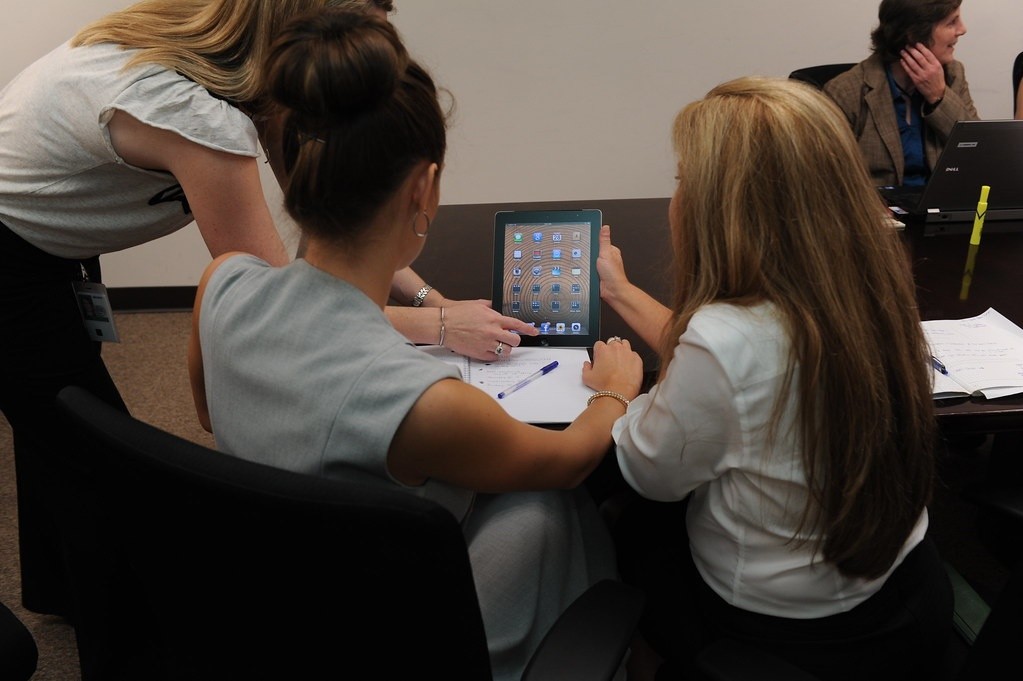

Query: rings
left=495, top=342, right=503, bottom=356
left=607, top=336, right=622, bottom=345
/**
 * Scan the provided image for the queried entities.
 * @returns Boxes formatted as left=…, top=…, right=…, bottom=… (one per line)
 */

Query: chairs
left=695, top=495, right=1023, bottom=681
left=56, top=383, right=637, bottom=681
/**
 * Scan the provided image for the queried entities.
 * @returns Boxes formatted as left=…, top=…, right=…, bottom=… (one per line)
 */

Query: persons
left=186, top=2, right=643, bottom=681
left=823, top=0, right=980, bottom=194
left=595, top=78, right=954, bottom=681
left=1012, top=51, right=1023, bottom=120
left=0, top=1, right=540, bottom=622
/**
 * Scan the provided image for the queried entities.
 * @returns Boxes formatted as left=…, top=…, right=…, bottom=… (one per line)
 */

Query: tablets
left=492, top=208, right=603, bottom=348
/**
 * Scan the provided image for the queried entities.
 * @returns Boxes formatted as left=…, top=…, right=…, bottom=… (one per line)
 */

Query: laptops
left=874, top=120, right=1023, bottom=222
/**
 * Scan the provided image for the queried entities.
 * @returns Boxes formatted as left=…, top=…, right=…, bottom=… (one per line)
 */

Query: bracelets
left=440, top=307, right=445, bottom=344
left=412, top=284, right=432, bottom=307
left=587, top=391, right=630, bottom=407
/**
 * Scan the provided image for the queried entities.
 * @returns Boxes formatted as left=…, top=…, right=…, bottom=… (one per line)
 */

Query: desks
left=295, top=197, right=1023, bottom=434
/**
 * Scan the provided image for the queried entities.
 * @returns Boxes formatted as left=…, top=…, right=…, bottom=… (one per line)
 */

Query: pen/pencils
left=926, top=353, right=948, bottom=376
left=498, top=360, right=559, bottom=400
